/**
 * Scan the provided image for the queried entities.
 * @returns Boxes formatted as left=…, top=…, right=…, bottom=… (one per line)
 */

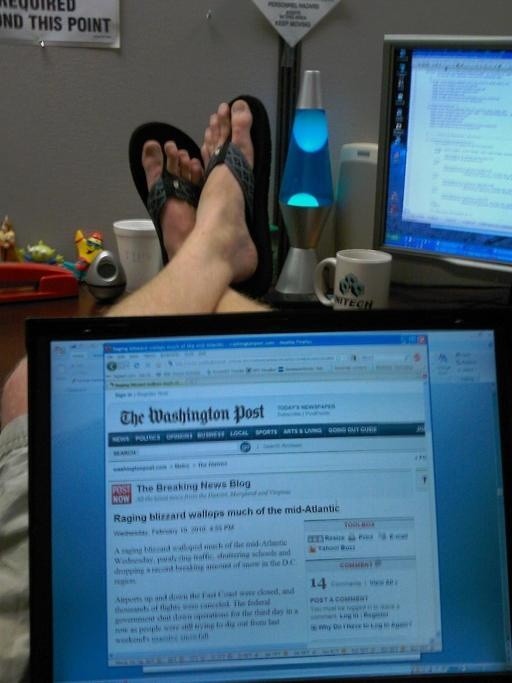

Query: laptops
left=23, top=306, right=512, bottom=682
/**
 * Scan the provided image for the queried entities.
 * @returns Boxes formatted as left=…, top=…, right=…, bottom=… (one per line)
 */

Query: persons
left=1, top=94, right=278, bottom=683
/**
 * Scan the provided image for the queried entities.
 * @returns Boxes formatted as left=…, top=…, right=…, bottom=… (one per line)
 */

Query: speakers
left=335, top=143, right=378, bottom=250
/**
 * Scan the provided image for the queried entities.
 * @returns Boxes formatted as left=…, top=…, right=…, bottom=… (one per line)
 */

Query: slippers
left=204, top=95, right=274, bottom=296
left=129, top=121, right=205, bottom=268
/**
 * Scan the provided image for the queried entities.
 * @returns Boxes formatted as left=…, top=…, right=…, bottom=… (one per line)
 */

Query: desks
left=0, top=253, right=512, bottom=407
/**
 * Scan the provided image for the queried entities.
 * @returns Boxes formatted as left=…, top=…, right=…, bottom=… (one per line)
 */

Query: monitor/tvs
left=371, top=34, right=512, bottom=274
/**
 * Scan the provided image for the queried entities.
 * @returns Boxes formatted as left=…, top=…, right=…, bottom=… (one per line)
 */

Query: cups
left=311, top=246, right=394, bottom=310
left=113, top=217, right=161, bottom=294
